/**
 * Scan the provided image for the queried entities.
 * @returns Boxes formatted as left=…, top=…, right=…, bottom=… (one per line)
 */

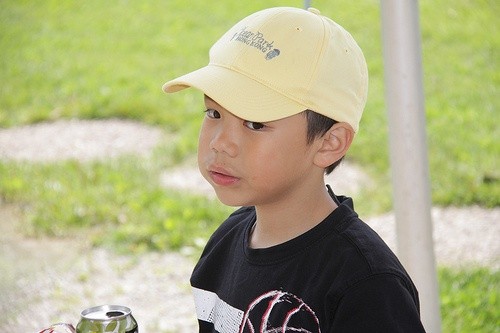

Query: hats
left=163, top=6, right=369, bottom=135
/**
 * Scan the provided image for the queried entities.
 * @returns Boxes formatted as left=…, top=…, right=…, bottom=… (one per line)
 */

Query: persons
left=163, top=6, right=426, bottom=333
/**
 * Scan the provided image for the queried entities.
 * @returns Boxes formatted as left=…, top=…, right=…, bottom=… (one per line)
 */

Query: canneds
left=75, top=305, right=139, bottom=333
